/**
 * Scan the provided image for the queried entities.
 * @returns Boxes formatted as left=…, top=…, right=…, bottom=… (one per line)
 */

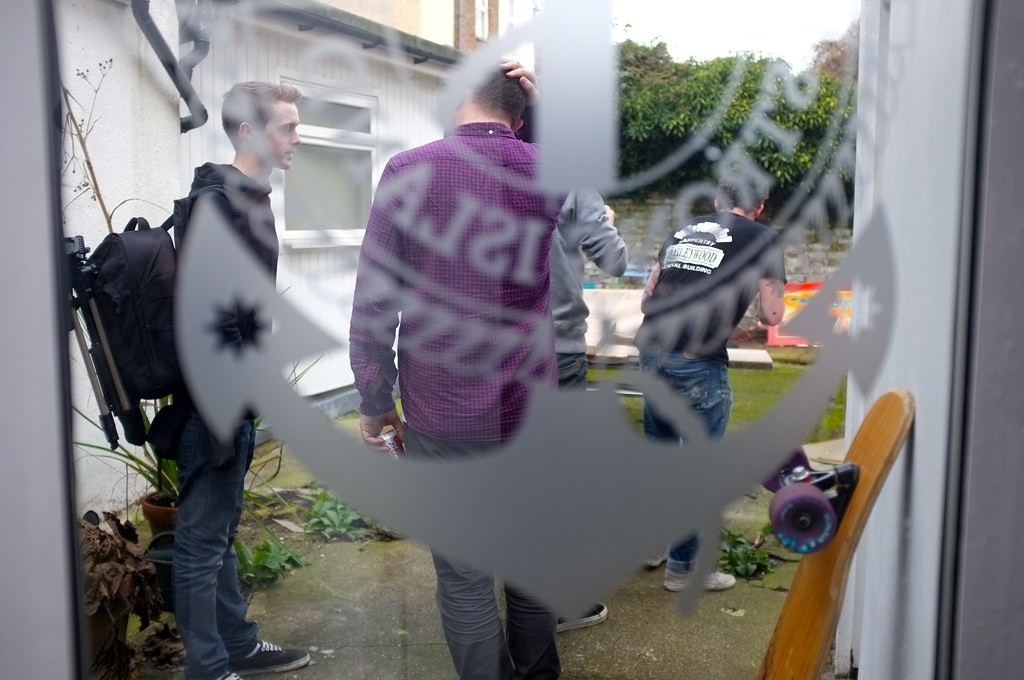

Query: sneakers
left=664, top=566, right=737, bottom=591
left=644, top=541, right=671, bottom=566
left=556, top=601, right=608, bottom=633
left=216, top=669, right=243, bottom=680
left=228, top=638, right=310, bottom=674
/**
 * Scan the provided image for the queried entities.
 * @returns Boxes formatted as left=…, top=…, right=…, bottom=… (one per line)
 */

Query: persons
left=554, top=186, right=627, bottom=633
left=172, top=80, right=310, bottom=680
left=349, top=58, right=586, bottom=680
left=632, top=166, right=788, bottom=591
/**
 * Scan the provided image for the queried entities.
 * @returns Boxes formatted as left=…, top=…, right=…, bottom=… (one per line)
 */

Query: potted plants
left=70, top=285, right=324, bottom=546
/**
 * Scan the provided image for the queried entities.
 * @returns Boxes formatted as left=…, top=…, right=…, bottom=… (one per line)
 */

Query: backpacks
left=57, top=182, right=251, bottom=450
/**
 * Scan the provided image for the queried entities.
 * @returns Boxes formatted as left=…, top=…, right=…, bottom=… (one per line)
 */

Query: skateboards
left=753, top=387, right=917, bottom=680
